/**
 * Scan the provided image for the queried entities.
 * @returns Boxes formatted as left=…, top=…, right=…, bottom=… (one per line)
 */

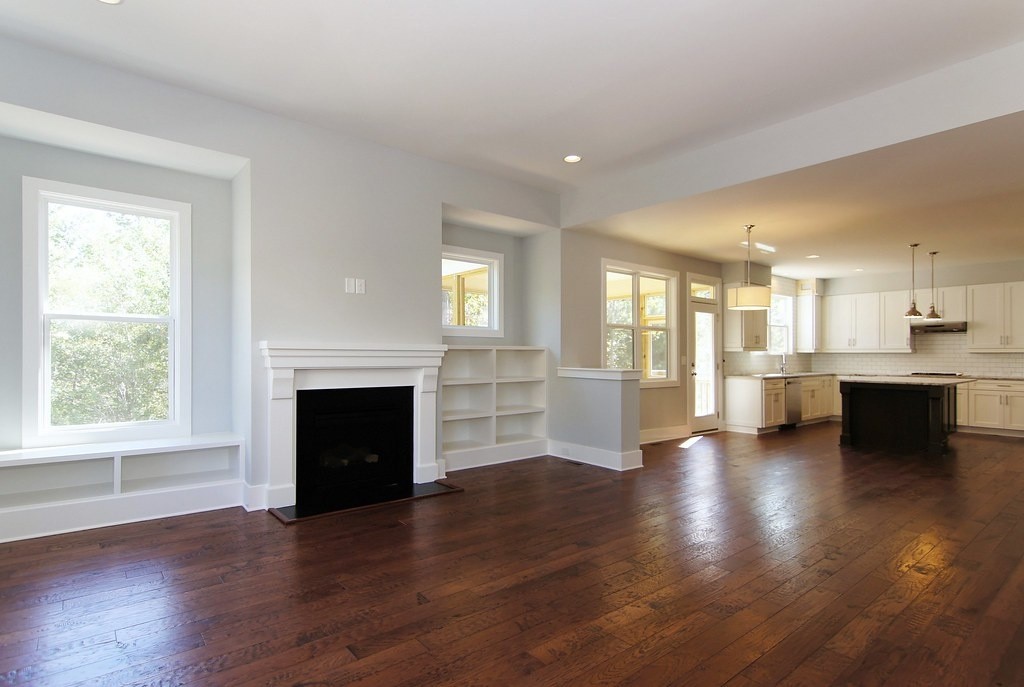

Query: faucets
left=780, top=354, right=789, bottom=376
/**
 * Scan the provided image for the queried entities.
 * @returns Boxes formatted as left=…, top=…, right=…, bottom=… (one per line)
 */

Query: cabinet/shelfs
left=440, top=345, right=552, bottom=474
left=721, top=283, right=1024, bottom=353
left=0, top=439, right=240, bottom=544
left=724, top=378, right=1024, bottom=442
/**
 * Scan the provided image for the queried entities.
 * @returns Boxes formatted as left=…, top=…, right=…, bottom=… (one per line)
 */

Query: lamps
left=928, top=250, right=941, bottom=320
left=905, top=243, right=923, bottom=318
left=728, top=223, right=771, bottom=310
